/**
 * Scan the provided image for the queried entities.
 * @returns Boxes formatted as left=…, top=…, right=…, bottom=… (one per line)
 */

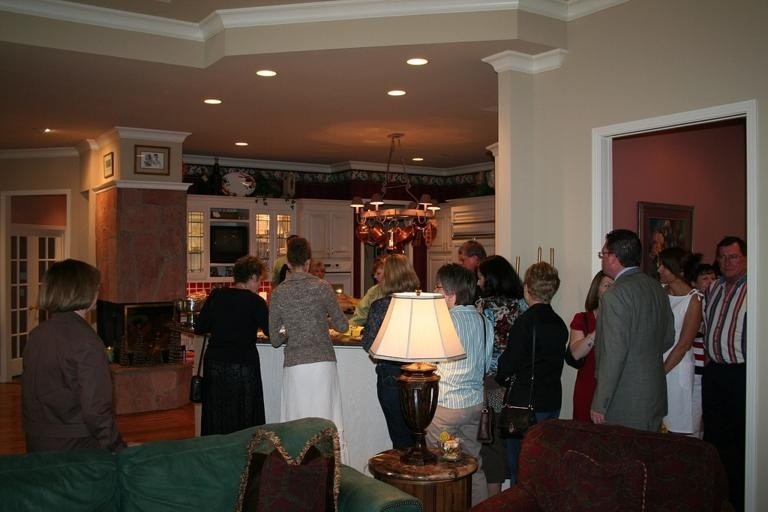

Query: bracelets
left=585, top=333, right=595, bottom=350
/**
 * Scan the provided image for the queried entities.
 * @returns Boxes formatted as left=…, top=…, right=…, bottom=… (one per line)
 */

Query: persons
left=364, top=254, right=421, bottom=449
left=460, top=240, right=486, bottom=271
left=272, top=236, right=299, bottom=287
left=650, top=236, right=748, bottom=506
left=590, top=229, right=676, bottom=433
left=415, top=261, right=488, bottom=507
left=569, top=270, right=616, bottom=423
left=269, top=237, right=350, bottom=462
left=496, top=261, right=566, bottom=486
left=475, top=256, right=525, bottom=498
left=22, top=260, right=125, bottom=454
left=144, top=154, right=162, bottom=169
left=307, top=261, right=326, bottom=277
left=351, top=253, right=387, bottom=325
left=193, top=255, right=269, bottom=435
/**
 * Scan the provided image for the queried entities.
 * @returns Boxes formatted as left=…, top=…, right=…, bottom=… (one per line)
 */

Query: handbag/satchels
left=499, top=404, right=538, bottom=440
left=189, top=375, right=205, bottom=403
left=564, top=341, right=587, bottom=370
left=476, top=405, right=496, bottom=444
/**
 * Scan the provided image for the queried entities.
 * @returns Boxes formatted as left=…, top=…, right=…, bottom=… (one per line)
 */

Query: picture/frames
left=638, top=201, right=696, bottom=283
left=134, top=144, right=170, bottom=176
left=102, top=152, right=114, bottom=178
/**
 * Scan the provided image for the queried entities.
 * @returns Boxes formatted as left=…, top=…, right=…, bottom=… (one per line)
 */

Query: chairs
left=467, top=418, right=731, bottom=512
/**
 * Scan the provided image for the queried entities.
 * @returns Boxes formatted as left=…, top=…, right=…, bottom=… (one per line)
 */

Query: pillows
left=236, top=426, right=342, bottom=512
left=561, top=451, right=648, bottom=511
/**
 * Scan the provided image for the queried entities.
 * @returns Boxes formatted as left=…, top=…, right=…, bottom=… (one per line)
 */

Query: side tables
left=370, top=448, right=478, bottom=512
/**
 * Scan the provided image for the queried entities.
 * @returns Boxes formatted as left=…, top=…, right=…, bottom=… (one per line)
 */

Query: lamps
left=367, top=288, right=467, bottom=465
left=349, top=132, right=440, bottom=251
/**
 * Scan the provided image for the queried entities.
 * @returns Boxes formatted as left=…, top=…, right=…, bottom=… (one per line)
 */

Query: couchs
left=0, top=417, right=422, bottom=511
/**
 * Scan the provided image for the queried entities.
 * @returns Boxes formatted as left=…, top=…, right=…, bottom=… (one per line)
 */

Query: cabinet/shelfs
left=427, top=203, right=451, bottom=253
left=453, top=240, right=495, bottom=267
left=296, top=199, right=353, bottom=262
left=452, top=195, right=495, bottom=239
left=427, top=252, right=452, bottom=292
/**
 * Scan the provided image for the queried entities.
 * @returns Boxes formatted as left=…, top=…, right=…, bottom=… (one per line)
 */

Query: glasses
left=716, top=254, right=746, bottom=262
left=598, top=251, right=615, bottom=259
